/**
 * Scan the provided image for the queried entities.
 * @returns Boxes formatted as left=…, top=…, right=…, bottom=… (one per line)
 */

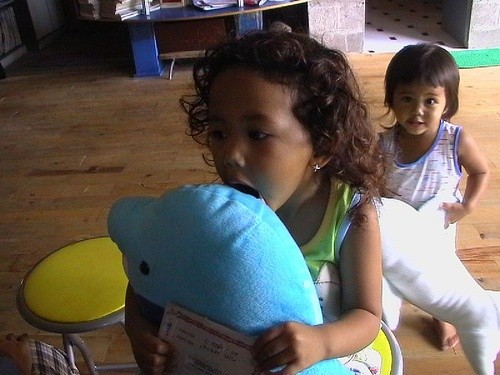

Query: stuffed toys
left=107, top=181, right=353, bottom=375
left=373, top=197, right=500, bottom=375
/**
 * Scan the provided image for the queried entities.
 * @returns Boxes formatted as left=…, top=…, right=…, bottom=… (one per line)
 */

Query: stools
left=368, top=320, right=403, bottom=375
left=15, top=236, right=134, bottom=375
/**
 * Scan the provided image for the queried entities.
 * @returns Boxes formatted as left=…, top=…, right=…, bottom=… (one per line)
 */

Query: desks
left=72, top=1, right=308, bottom=78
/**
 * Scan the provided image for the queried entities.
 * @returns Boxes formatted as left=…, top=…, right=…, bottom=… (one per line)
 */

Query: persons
left=372, top=43, right=490, bottom=352
left=178, top=31, right=395, bottom=375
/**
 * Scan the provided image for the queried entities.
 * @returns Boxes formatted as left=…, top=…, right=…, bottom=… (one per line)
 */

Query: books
left=112, top=0, right=160, bottom=22
